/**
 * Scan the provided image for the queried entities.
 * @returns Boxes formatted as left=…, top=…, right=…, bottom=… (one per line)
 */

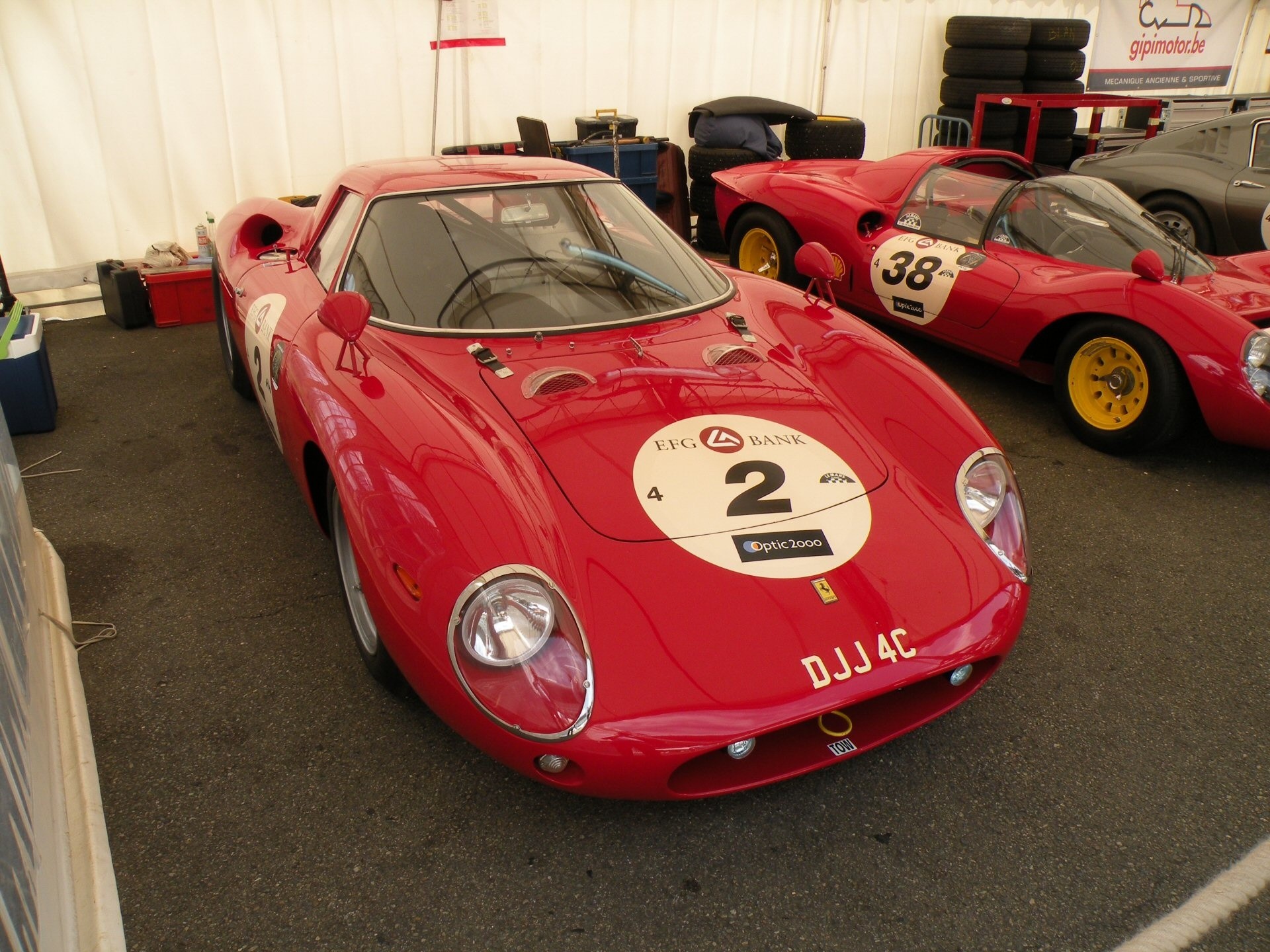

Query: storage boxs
left=143, top=263, right=218, bottom=329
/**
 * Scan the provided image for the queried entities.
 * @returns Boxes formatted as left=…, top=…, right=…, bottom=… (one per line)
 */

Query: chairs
left=356, top=206, right=493, bottom=338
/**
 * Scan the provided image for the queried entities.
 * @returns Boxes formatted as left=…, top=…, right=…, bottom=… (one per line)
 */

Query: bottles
left=195, top=223, right=211, bottom=258
left=205, top=211, right=217, bottom=257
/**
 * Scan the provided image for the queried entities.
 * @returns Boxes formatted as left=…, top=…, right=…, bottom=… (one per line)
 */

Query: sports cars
left=1068, top=105, right=1270, bottom=253
left=707, top=149, right=1270, bottom=458
left=206, top=155, right=1026, bottom=803
